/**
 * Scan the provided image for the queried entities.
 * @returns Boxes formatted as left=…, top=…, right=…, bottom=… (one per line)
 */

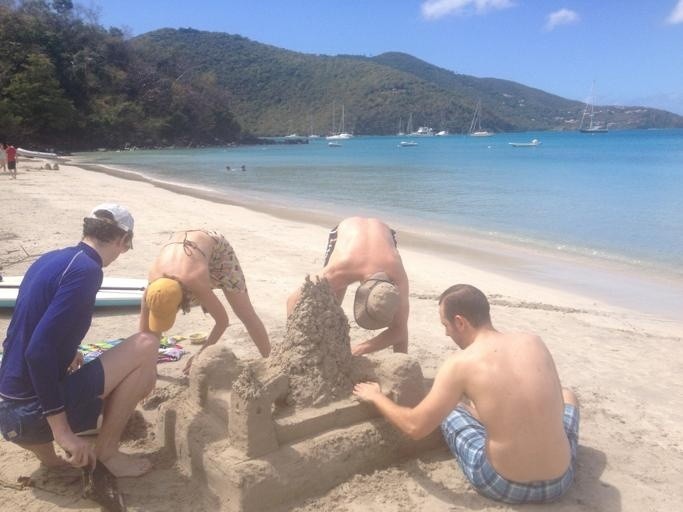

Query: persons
left=1, top=202, right=161, bottom=479
left=0, top=142, right=4, bottom=175
left=350, top=282, right=579, bottom=502
left=4, top=140, right=17, bottom=179
left=283, top=216, right=411, bottom=359
left=139, top=228, right=269, bottom=375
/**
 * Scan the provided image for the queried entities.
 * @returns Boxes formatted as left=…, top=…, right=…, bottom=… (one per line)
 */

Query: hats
left=83, top=203, right=134, bottom=250
left=145, top=278, right=183, bottom=332
left=353, top=280, right=401, bottom=330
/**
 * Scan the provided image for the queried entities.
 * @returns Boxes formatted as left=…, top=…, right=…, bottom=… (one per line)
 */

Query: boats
left=410, top=126, right=449, bottom=138
left=509, top=139, right=543, bottom=148
left=16, top=148, right=57, bottom=159
left=328, top=142, right=343, bottom=147
left=401, top=142, right=418, bottom=146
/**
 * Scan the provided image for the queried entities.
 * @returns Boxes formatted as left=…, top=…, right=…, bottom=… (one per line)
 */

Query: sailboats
left=469, top=97, right=494, bottom=137
left=574, top=80, right=609, bottom=133
left=284, top=98, right=354, bottom=140
